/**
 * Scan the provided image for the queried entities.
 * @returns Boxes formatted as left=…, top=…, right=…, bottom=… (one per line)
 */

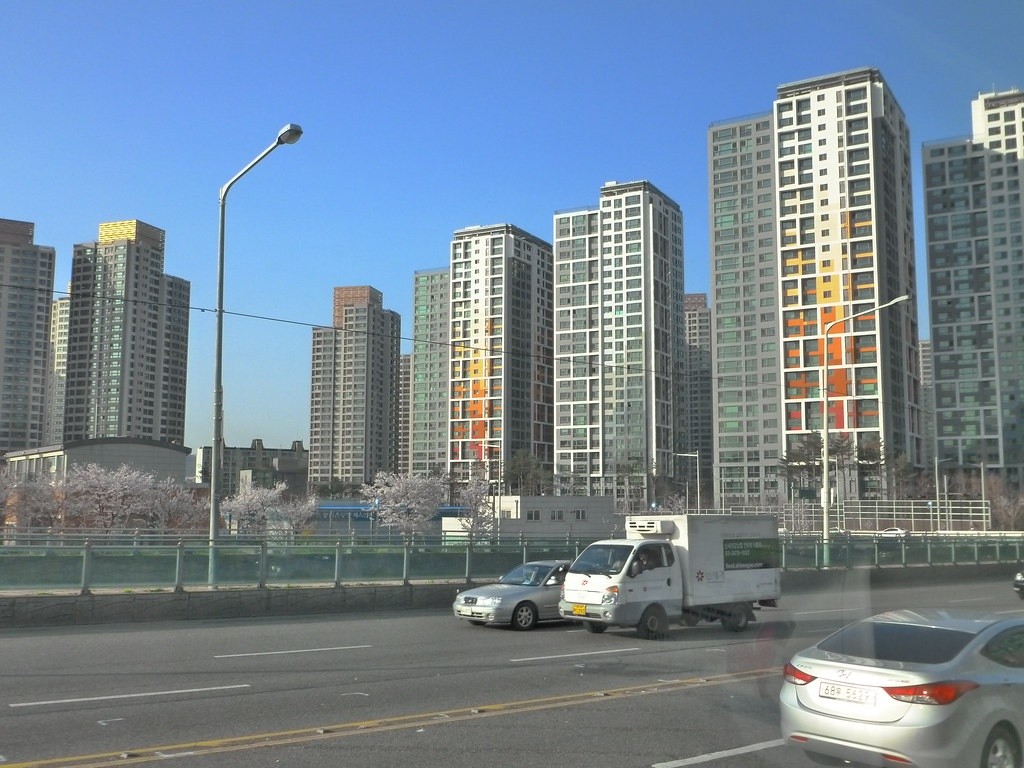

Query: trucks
left=557, top=511, right=781, bottom=641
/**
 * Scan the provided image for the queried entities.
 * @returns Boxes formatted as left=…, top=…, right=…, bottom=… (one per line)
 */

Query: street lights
left=820, top=293, right=911, bottom=567
left=206, top=121, right=305, bottom=588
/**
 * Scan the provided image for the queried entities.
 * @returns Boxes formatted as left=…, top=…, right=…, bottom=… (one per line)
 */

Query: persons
left=638, top=548, right=655, bottom=574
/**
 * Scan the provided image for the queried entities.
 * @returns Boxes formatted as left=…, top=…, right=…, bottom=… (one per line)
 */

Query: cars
left=875, top=527, right=910, bottom=537
left=776, top=604, right=1024, bottom=768
left=453, top=558, right=582, bottom=630
left=828, top=526, right=847, bottom=533
